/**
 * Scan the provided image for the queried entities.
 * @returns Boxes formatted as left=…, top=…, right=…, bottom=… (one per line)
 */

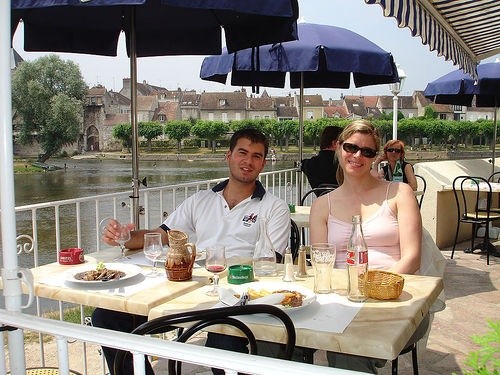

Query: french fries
left=248, top=288, right=296, bottom=305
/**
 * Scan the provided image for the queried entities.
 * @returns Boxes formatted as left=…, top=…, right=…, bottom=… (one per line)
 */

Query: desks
left=445, top=181, right=500, bottom=193
left=289, top=205, right=311, bottom=227
left=0, top=246, right=443, bottom=375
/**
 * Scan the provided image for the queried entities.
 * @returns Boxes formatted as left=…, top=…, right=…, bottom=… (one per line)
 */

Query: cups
left=309, top=243, right=336, bottom=294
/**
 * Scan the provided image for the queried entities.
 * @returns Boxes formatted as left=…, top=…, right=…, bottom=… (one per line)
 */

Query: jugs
left=164, top=229, right=197, bottom=282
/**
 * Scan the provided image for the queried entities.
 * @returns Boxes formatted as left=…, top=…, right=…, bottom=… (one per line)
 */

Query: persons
left=92, top=129, right=291, bottom=375
left=372, top=140, right=416, bottom=191
left=302, top=126, right=343, bottom=197
left=252, top=120, right=422, bottom=375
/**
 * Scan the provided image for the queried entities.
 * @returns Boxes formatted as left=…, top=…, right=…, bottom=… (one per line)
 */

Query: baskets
left=358, top=270, right=404, bottom=300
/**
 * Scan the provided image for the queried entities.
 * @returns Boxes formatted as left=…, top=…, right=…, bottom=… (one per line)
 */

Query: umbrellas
left=201, top=24, right=399, bottom=205
left=11, top=0, right=299, bottom=231
left=424, top=62, right=500, bottom=178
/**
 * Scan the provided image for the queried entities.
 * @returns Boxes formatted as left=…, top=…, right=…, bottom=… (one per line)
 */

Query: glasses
left=342, top=142, right=378, bottom=158
left=387, top=149, right=401, bottom=153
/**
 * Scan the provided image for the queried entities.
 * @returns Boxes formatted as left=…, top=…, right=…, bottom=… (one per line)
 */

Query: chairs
left=112, top=304, right=297, bottom=375
left=414, top=174, right=426, bottom=209
left=478, top=171, right=500, bottom=213
left=451, top=176, right=500, bottom=264
left=276, top=218, right=300, bottom=264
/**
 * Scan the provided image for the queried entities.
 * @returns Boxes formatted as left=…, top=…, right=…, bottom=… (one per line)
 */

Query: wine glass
left=144, top=232, right=163, bottom=275
left=204, top=244, right=227, bottom=296
left=112, top=224, right=132, bottom=263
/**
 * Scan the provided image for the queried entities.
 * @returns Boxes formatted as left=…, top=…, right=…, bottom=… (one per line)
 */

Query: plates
left=63, top=261, right=141, bottom=282
left=220, top=281, right=317, bottom=317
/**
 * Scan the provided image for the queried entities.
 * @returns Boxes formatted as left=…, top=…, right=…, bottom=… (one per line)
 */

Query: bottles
left=251, top=219, right=277, bottom=277
left=345, top=215, right=370, bottom=302
left=282, top=244, right=309, bottom=282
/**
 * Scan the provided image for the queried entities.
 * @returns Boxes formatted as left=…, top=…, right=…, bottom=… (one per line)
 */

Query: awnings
left=364, top=0, right=500, bottom=83
